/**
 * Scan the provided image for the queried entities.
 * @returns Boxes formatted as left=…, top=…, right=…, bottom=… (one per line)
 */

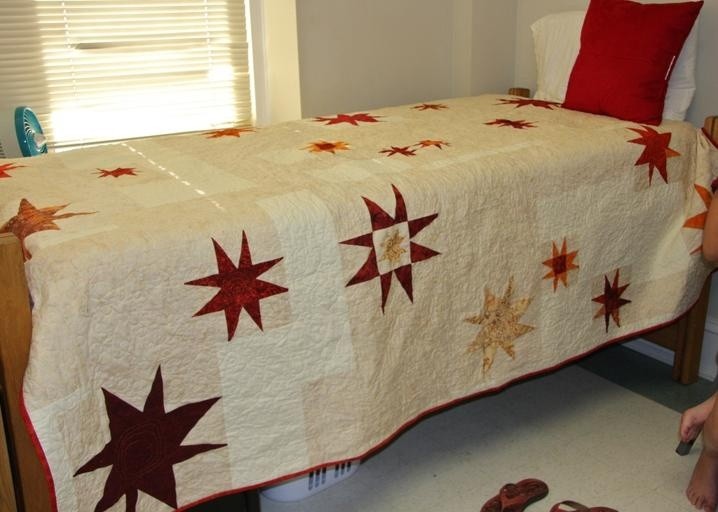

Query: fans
left=0, top=107, right=49, bottom=158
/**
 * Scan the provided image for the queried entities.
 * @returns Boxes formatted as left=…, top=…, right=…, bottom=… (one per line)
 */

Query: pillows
left=528, top=8, right=700, bottom=122
left=559, top=0, right=705, bottom=127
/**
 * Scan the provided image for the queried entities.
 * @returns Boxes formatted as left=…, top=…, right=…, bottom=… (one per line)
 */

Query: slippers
left=478, top=477, right=550, bottom=512
left=548, top=499, right=619, bottom=512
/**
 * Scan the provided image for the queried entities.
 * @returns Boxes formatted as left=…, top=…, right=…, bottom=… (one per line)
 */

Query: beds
left=0, top=1, right=717, bottom=512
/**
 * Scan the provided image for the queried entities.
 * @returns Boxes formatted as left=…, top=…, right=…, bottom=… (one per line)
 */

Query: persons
left=677, top=181, right=717, bottom=511
